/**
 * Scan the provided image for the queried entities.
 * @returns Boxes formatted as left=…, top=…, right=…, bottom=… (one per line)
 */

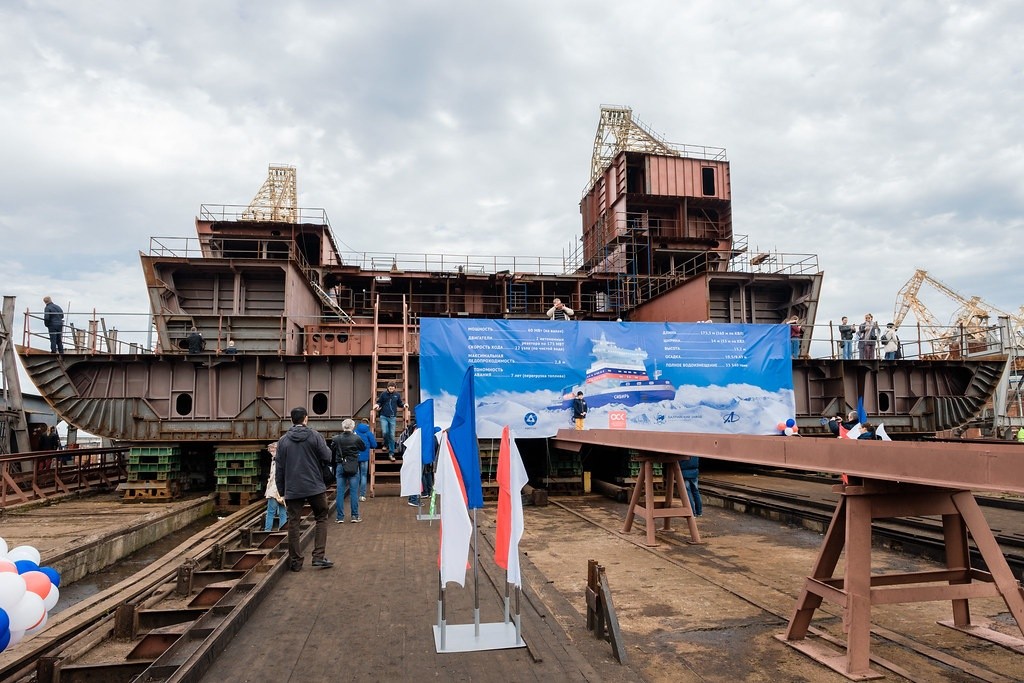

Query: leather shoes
left=312, top=558, right=335, bottom=567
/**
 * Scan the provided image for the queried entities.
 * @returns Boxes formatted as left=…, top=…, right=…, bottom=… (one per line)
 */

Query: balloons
left=776, top=418, right=798, bottom=436
left=0, top=536, right=61, bottom=654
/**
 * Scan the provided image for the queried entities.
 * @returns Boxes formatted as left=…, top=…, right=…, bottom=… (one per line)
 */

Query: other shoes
left=335, top=518, right=344, bottom=523
left=351, top=515, right=363, bottom=523
left=408, top=500, right=424, bottom=507
left=381, top=443, right=388, bottom=450
left=420, top=492, right=428, bottom=499
left=696, top=514, right=702, bottom=517
left=389, top=453, right=396, bottom=461
left=359, top=496, right=367, bottom=502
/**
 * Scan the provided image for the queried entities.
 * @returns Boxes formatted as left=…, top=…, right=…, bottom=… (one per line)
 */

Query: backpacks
left=395, top=424, right=416, bottom=454
left=881, top=330, right=892, bottom=345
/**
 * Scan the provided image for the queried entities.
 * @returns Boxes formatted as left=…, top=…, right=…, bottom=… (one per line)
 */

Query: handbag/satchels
left=341, top=460, right=357, bottom=474
left=321, top=463, right=337, bottom=487
left=840, top=341, right=845, bottom=348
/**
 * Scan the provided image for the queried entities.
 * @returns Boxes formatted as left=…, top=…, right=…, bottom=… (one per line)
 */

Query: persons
left=275, top=407, right=333, bottom=572
left=857, top=422, right=882, bottom=440
left=677, top=455, right=702, bottom=518
left=262, top=442, right=287, bottom=532
left=185, top=326, right=206, bottom=353
left=43, top=296, right=64, bottom=354
left=32, top=425, right=60, bottom=475
left=786, top=315, right=802, bottom=359
left=373, top=380, right=409, bottom=461
left=353, top=418, right=377, bottom=501
left=856, top=313, right=901, bottom=359
left=828, top=411, right=860, bottom=438
left=396, top=421, right=438, bottom=507
left=574, top=391, right=587, bottom=431
left=331, top=419, right=366, bottom=523
left=1017, top=426, right=1024, bottom=443
left=839, top=316, right=856, bottom=360
left=219, top=340, right=237, bottom=354
left=546, top=298, right=574, bottom=320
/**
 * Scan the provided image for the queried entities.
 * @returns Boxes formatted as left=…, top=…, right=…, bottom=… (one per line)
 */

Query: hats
left=362, top=418, right=369, bottom=424
